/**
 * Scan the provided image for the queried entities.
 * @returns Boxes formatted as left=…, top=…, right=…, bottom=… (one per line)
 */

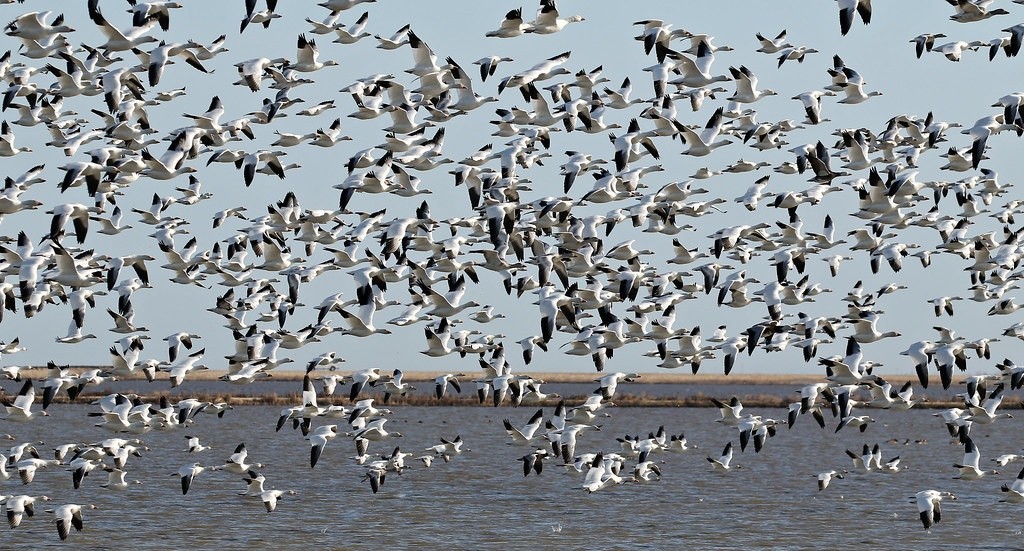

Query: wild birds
left=0, top=1, right=1024, bottom=551
